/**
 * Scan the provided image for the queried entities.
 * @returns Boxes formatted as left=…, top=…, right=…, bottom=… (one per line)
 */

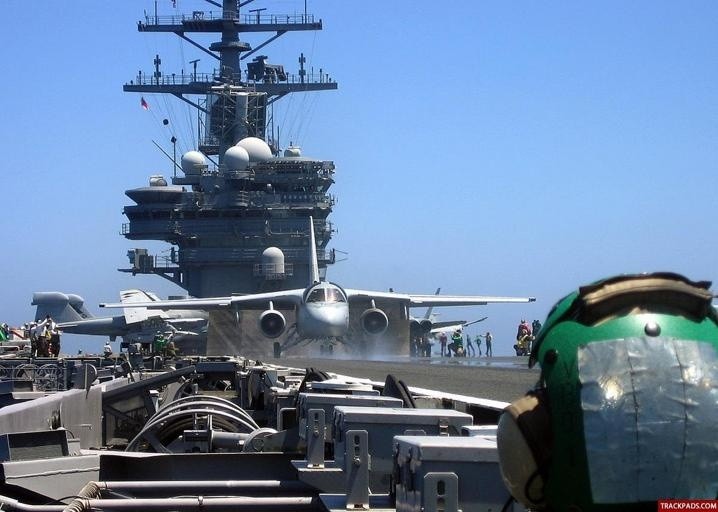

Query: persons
left=515, top=329, right=533, bottom=355
left=532, top=319, right=541, bottom=346
left=168, top=341, right=179, bottom=356
left=466, top=334, right=475, bottom=356
left=28, top=318, right=63, bottom=358
left=474, top=335, right=482, bottom=355
left=413, top=330, right=463, bottom=357
left=516, top=319, right=531, bottom=353
left=480, top=332, right=493, bottom=355
left=1, top=321, right=9, bottom=339
left=104, top=341, right=112, bottom=357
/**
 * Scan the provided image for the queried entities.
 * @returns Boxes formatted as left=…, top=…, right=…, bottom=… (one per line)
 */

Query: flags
left=141, top=97, right=148, bottom=110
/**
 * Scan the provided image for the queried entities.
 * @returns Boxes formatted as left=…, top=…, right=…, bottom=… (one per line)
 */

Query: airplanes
left=30, top=291, right=205, bottom=351
left=389, top=286, right=489, bottom=358
left=98, top=215, right=537, bottom=358
